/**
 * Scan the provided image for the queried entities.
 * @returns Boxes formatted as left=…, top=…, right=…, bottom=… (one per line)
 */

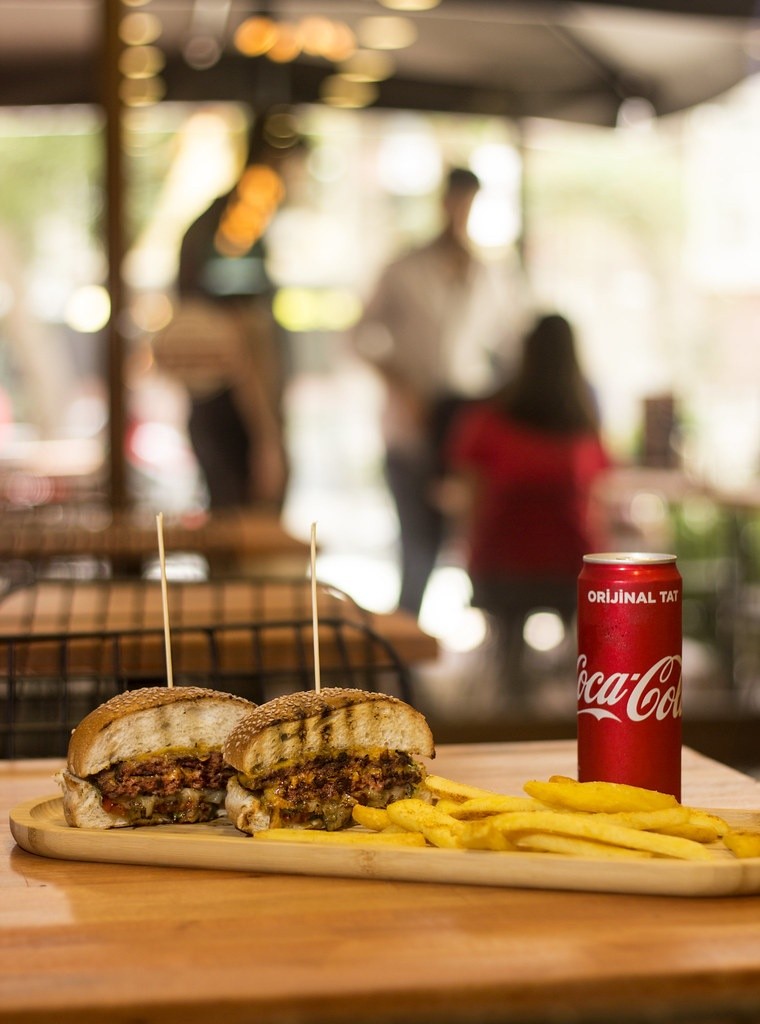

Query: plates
left=10, top=791, right=760, bottom=897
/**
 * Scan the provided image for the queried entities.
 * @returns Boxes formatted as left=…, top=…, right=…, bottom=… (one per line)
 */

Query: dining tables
left=1, top=737, right=760, bottom=1023
left=1, top=498, right=441, bottom=757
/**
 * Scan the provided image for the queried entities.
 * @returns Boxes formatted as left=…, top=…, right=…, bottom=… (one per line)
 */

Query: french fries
left=252, top=775, right=760, bottom=862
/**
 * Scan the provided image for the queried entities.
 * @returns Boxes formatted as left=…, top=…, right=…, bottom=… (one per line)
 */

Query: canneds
left=574, top=549, right=683, bottom=803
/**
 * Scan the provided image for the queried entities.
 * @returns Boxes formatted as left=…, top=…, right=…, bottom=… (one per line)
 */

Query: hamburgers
left=56, top=687, right=435, bottom=840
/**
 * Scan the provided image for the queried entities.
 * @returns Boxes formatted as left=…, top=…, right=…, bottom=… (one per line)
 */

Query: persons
left=350, top=164, right=552, bottom=633
left=175, top=115, right=317, bottom=582
left=428, top=309, right=629, bottom=648
